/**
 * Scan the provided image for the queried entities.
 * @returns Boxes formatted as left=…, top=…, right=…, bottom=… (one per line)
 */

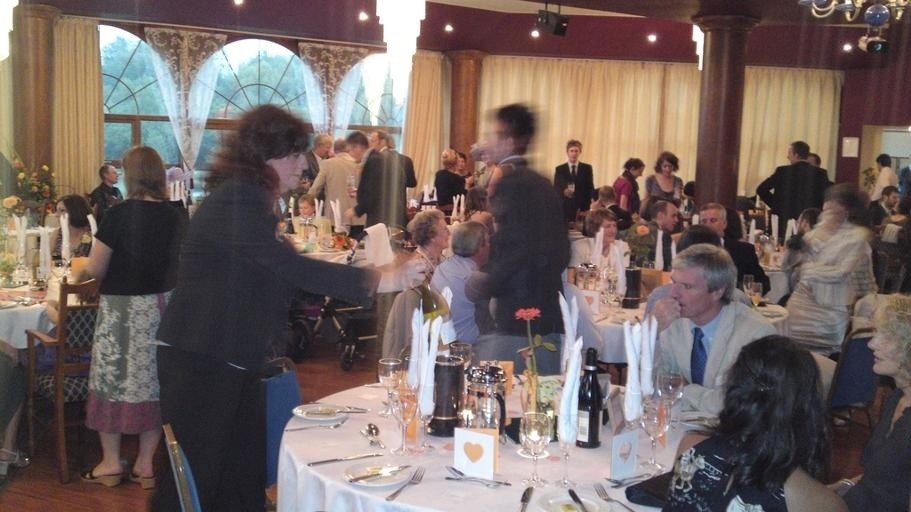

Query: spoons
left=358, top=421, right=384, bottom=452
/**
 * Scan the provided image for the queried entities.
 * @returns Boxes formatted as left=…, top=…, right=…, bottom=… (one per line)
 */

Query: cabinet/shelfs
left=859, top=124, right=911, bottom=198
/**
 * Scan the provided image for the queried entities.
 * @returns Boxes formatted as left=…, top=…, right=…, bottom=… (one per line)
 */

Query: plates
left=569, top=228, right=585, bottom=240
left=292, top=401, right=348, bottom=421
left=346, top=465, right=410, bottom=486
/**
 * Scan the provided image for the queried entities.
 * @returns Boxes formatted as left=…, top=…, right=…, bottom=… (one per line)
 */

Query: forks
left=297, top=423, right=345, bottom=432
left=387, top=467, right=425, bottom=505
left=594, top=483, right=638, bottom=512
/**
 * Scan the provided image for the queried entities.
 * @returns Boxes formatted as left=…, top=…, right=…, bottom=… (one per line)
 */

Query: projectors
left=858, top=36, right=890, bottom=55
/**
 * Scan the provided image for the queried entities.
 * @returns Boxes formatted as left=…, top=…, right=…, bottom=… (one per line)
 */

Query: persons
left=829, top=292, right=911, bottom=512
left=143, top=103, right=419, bottom=510
left=5, top=254, right=94, bottom=377
left=660, top=333, right=858, bottom=512
left=86, top=160, right=127, bottom=232
left=167, top=166, right=185, bottom=184
left=46, top=193, right=100, bottom=265
left=68, top=145, right=189, bottom=490
left=0, top=350, right=32, bottom=479
left=463, top=102, right=579, bottom=382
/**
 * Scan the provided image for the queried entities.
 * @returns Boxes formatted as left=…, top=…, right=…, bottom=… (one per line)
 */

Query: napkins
left=557, top=336, right=583, bottom=443
left=417, top=315, right=442, bottom=416
left=640, top=313, right=658, bottom=395
left=3, top=193, right=98, bottom=349
left=405, top=299, right=424, bottom=390
left=558, top=291, right=578, bottom=370
left=623, top=320, right=643, bottom=421
left=441, top=285, right=453, bottom=309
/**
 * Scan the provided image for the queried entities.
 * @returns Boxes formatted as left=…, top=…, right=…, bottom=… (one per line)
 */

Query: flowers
left=5, top=143, right=60, bottom=218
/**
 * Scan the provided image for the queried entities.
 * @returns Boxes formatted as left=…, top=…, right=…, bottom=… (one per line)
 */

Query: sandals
left=78, top=467, right=124, bottom=488
left=127, top=469, right=157, bottom=491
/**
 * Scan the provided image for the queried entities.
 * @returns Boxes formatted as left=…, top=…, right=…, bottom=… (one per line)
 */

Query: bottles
left=750, top=231, right=786, bottom=268
left=570, top=261, right=622, bottom=324
left=279, top=211, right=351, bottom=255
left=577, top=347, right=604, bottom=447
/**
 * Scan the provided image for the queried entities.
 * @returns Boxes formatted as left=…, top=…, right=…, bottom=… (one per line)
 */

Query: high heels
left=0, top=445, right=33, bottom=475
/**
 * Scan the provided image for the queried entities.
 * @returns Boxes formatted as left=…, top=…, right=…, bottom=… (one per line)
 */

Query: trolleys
left=289, top=222, right=410, bottom=370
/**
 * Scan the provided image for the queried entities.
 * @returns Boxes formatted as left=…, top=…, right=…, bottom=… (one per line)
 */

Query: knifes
left=305, top=452, right=383, bottom=468
left=518, top=487, right=536, bottom=512
left=443, top=466, right=512, bottom=491
left=348, top=467, right=404, bottom=485
left=568, top=489, right=588, bottom=511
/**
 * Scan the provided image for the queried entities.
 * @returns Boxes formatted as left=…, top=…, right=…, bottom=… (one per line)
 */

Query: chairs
left=278, top=192, right=466, bottom=367
left=24, top=275, right=100, bottom=484
left=808, top=327, right=878, bottom=455
left=161, top=423, right=200, bottom=504
left=474, top=334, right=562, bottom=375
left=562, top=192, right=910, bottom=363
left=263, top=359, right=301, bottom=503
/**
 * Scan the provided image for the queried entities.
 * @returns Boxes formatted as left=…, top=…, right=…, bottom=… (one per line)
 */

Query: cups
left=430, top=354, right=462, bottom=436
left=449, top=340, right=473, bottom=361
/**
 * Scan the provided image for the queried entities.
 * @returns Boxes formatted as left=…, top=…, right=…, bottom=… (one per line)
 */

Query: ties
left=570, top=165, right=577, bottom=177
left=688, top=326, right=708, bottom=388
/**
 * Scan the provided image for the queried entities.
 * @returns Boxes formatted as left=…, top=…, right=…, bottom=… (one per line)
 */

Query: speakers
left=536, top=10, right=568, bottom=37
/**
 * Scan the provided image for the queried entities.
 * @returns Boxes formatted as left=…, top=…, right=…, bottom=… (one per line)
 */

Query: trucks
left=191, top=169, right=214, bottom=206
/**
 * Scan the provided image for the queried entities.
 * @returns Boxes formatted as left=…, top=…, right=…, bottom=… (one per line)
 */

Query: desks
left=277, top=370, right=745, bottom=504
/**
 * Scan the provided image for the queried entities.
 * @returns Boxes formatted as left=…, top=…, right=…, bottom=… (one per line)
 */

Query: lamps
left=798, top=0, right=911, bottom=24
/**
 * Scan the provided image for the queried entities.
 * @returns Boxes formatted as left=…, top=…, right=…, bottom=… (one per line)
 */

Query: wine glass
left=0, top=241, right=69, bottom=305
left=742, top=274, right=763, bottom=310
left=377, top=355, right=425, bottom=456
left=639, top=367, right=687, bottom=473
left=518, top=414, right=581, bottom=492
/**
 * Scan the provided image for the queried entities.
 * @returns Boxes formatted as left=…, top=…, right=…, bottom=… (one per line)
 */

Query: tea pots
left=465, top=361, right=506, bottom=445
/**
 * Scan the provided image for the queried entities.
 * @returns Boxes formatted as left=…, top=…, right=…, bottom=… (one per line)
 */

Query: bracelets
left=837, top=472, right=857, bottom=495
